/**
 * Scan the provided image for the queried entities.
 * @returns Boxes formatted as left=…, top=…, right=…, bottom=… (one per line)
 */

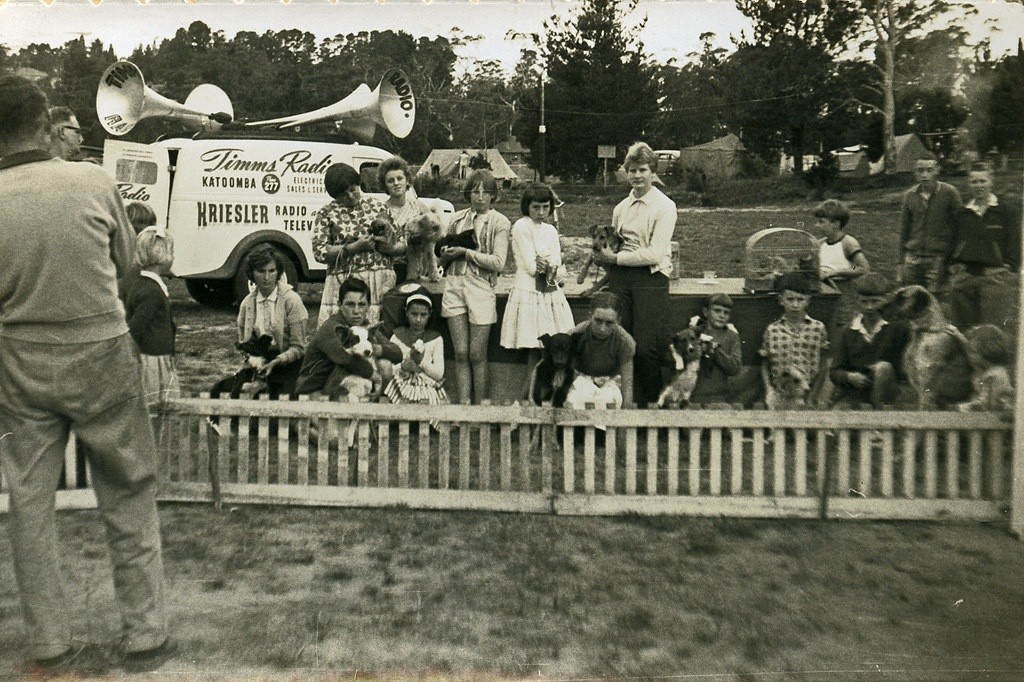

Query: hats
left=920, top=150, right=937, bottom=160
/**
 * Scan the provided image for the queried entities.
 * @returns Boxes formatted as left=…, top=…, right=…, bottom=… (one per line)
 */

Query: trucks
left=98, top=133, right=457, bottom=310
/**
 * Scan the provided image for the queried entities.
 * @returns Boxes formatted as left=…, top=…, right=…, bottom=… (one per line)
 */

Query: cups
left=703, top=270, right=716, bottom=283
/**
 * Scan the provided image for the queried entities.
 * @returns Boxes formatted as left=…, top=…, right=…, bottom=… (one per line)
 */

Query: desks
left=380, top=276, right=609, bottom=363
left=669, top=278, right=843, bottom=367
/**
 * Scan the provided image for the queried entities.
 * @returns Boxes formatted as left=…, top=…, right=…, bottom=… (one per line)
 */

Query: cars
left=652, top=149, right=681, bottom=173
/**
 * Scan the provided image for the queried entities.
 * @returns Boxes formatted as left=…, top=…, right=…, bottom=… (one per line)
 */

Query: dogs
left=767, top=364, right=811, bottom=446
left=369, top=212, right=447, bottom=282
left=211, top=334, right=281, bottom=424
left=403, top=339, right=425, bottom=384
left=322, top=320, right=385, bottom=447
left=877, top=285, right=974, bottom=465
left=636, top=326, right=719, bottom=410
left=529, top=333, right=582, bottom=453
left=577, top=224, right=624, bottom=297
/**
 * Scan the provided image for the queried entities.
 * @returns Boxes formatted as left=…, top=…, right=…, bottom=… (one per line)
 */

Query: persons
left=0, top=75, right=178, bottom=675
left=48, top=105, right=83, bottom=160
left=80, top=141, right=1022, bottom=471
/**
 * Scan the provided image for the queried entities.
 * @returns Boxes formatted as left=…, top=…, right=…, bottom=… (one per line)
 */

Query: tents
left=827, top=142, right=868, bottom=177
left=872, top=133, right=938, bottom=175
left=414, top=148, right=517, bottom=188
left=681, top=134, right=767, bottom=180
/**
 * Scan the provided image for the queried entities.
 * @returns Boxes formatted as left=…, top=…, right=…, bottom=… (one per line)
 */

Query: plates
left=698, top=281, right=720, bottom=285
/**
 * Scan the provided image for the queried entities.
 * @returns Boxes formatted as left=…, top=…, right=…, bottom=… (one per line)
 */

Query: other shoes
left=206, top=416, right=220, bottom=435
left=35, top=646, right=75, bottom=668
left=127, top=634, right=182, bottom=672
